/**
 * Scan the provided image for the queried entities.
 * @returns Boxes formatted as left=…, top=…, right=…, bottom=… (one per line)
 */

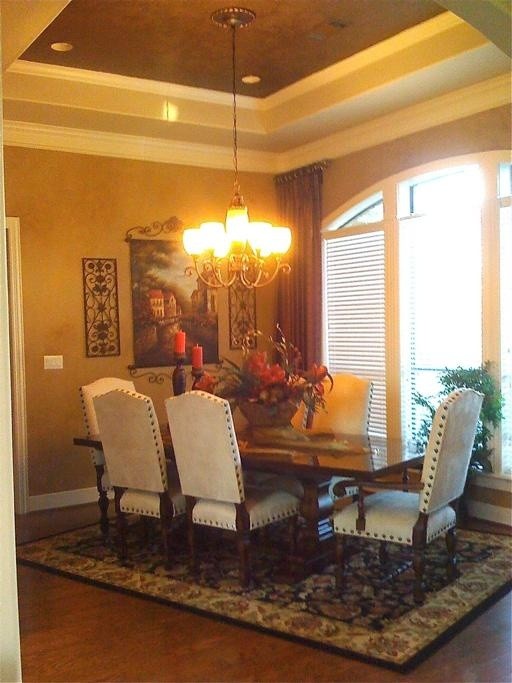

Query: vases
left=238, top=402, right=308, bottom=441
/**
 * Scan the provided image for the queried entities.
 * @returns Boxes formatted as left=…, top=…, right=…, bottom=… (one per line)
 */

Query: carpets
left=17, top=511, right=512, bottom=675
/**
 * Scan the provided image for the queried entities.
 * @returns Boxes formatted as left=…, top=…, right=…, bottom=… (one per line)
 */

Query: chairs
left=79, top=377, right=151, bottom=531
left=312, top=373, right=375, bottom=516
left=164, top=390, right=301, bottom=587
left=93, top=388, right=189, bottom=558
left=333, top=389, right=483, bottom=596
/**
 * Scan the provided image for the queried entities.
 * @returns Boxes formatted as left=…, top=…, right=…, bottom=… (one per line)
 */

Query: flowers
left=196, top=325, right=335, bottom=429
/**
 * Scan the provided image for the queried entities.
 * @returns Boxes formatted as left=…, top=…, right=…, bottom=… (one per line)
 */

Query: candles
left=175, top=329, right=185, bottom=353
left=192, top=344, right=202, bottom=369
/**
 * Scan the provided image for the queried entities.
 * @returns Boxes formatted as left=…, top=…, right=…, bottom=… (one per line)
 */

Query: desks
left=74, top=421, right=426, bottom=584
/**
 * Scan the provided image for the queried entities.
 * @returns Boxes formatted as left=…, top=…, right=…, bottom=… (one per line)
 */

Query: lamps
left=182, top=8, right=293, bottom=289
